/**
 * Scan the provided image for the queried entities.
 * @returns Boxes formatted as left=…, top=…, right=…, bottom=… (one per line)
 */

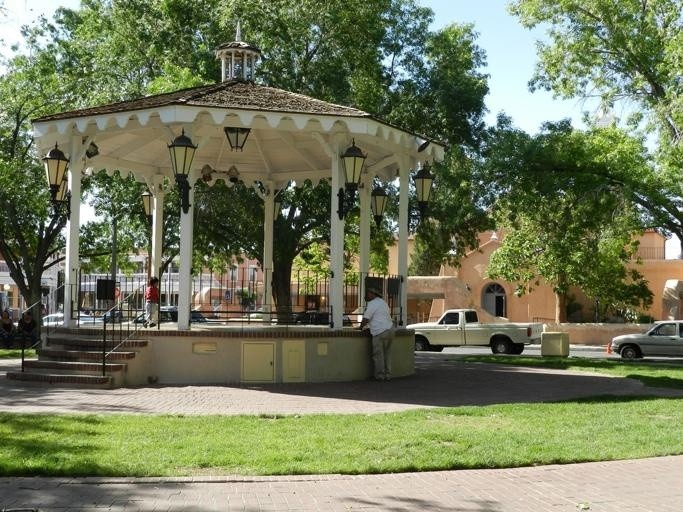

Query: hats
left=365, top=284, right=384, bottom=297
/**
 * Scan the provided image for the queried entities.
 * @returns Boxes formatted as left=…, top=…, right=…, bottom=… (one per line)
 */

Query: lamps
left=370, top=184, right=389, bottom=226
left=406, top=164, right=435, bottom=229
left=140, top=188, right=152, bottom=225
left=40, top=140, right=71, bottom=221
left=165, top=127, right=198, bottom=214
left=337, top=136, right=368, bottom=220
left=223, top=127, right=251, bottom=152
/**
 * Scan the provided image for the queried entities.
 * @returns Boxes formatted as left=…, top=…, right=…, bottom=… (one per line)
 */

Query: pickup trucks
left=406, top=309, right=543, bottom=355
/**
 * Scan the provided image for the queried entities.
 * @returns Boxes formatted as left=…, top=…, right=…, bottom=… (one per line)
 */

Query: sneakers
left=365, top=375, right=392, bottom=384
left=143, top=321, right=156, bottom=328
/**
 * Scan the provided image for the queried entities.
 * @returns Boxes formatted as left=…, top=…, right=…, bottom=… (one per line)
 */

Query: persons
left=0, top=310, right=16, bottom=350
left=141, top=276, right=160, bottom=328
left=354, top=285, right=393, bottom=382
left=16, top=311, right=38, bottom=348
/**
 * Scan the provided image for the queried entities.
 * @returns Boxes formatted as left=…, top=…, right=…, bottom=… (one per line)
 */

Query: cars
left=13, top=312, right=103, bottom=327
left=610, top=320, right=683, bottom=358
left=293, top=311, right=353, bottom=327
left=133, top=306, right=207, bottom=324
